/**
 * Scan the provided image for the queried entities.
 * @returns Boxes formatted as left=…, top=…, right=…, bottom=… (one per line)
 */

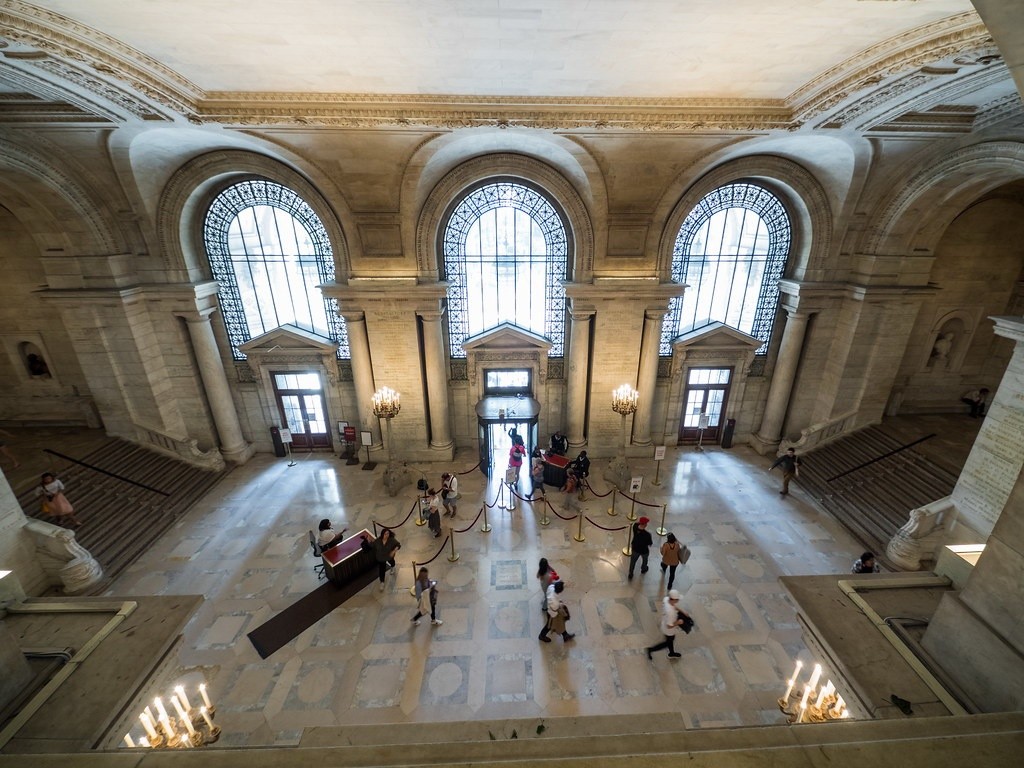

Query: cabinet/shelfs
left=541, top=449, right=572, bottom=488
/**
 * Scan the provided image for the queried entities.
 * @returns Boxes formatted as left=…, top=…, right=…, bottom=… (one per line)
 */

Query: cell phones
left=431, top=581, right=436, bottom=585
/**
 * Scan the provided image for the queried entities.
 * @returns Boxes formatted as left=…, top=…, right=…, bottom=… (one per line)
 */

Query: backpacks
left=513, top=446, right=521, bottom=460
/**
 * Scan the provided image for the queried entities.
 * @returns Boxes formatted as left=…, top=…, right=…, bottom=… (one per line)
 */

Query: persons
left=538, top=580, right=575, bottom=642
left=645, top=590, right=688, bottom=660
left=34, top=472, right=82, bottom=526
left=410, top=567, right=443, bottom=626
left=660, top=532, right=680, bottom=590
left=628, top=517, right=653, bottom=582
left=318, top=519, right=343, bottom=553
left=768, top=447, right=802, bottom=495
left=367, top=528, right=401, bottom=591
left=525, top=446, right=546, bottom=500
left=960, top=388, right=990, bottom=419
left=559, top=468, right=582, bottom=512
left=441, top=473, right=458, bottom=519
left=28, top=353, right=46, bottom=375
left=508, top=427, right=526, bottom=492
left=558, top=451, right=590, bottom=492
left=548, top=431, right=569, bottom=456
left=424, top=488, right=441, bottom=538
left=850, top=552, right=880, bottom=574
left=934, top=333, right=954, bottom=360
left=537, top=558, right=560, bottom=611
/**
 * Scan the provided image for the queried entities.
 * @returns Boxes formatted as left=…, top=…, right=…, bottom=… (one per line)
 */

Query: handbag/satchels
left=441, top=484, right=449, bottom=499
left=562, top=605, right=570, bottom=620
left=430, top=590, right=438, bottom=601
left=506, top=465, right=516, bottom=484
left=676, top=611, right=694, bottom=634
left=41, top=484, right=55, bottom=502
left=677, top=540, right=691, bottom=564
left=795, top=456, right=799, bottom=477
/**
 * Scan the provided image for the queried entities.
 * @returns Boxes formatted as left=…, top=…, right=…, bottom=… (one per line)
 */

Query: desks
left=322, top=527, right=378, bottom=579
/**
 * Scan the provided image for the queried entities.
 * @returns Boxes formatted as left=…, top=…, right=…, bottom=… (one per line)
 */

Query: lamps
left=121, top=682, right=222, bottom=747
left=935, top=543, right=986, bottom=588
left=775, top=660, right=850, bottom=722
left=603, top=382, right=643, bottom=492
left=369, top=385, right=414, bottom=498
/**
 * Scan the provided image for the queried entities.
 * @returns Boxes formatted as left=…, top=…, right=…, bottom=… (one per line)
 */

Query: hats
left=669, top=590, right=683, bottom=599
left=639, top=516, right=650, bottom=524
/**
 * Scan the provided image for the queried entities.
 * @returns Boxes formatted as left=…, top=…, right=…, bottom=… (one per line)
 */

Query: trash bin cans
left=270, top=427, right=286, bottom=457
left=721, top=419, right=735, bottom=448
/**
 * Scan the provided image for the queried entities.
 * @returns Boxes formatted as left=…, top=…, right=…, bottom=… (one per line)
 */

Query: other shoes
left=515, top=485, right=517, bottom=490
left=668, top=652, right=681, bottom=657
left=628, top=574, right=633, bottom=579
left=435, top=532, right=441, bottom=538
left=564, top=633, right=575, bottom=642
left=451, top=513, right=457, bottom=519
left=74, top=520, right=82, bottom=525
left=58, top=520, right=63, bottom=526
left=641, top=566, right=649, bottom=574
left=431, top=619, right=443, bottom=625
left=410, top=619, right=420, bottom=626
left=379, top=582, right=385, bottom=591
left=539, top=635, right=551, bottom=642
left=443, top=511, right=451, bottom=516
left=647, top=647, right=653, bottom=659
left=390, top=565, right=396, bottom=574
left=525, top=494, right=531, bottom=499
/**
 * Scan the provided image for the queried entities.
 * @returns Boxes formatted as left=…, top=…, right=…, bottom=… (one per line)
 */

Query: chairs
left=308, top=529, right=326, bottom=580
